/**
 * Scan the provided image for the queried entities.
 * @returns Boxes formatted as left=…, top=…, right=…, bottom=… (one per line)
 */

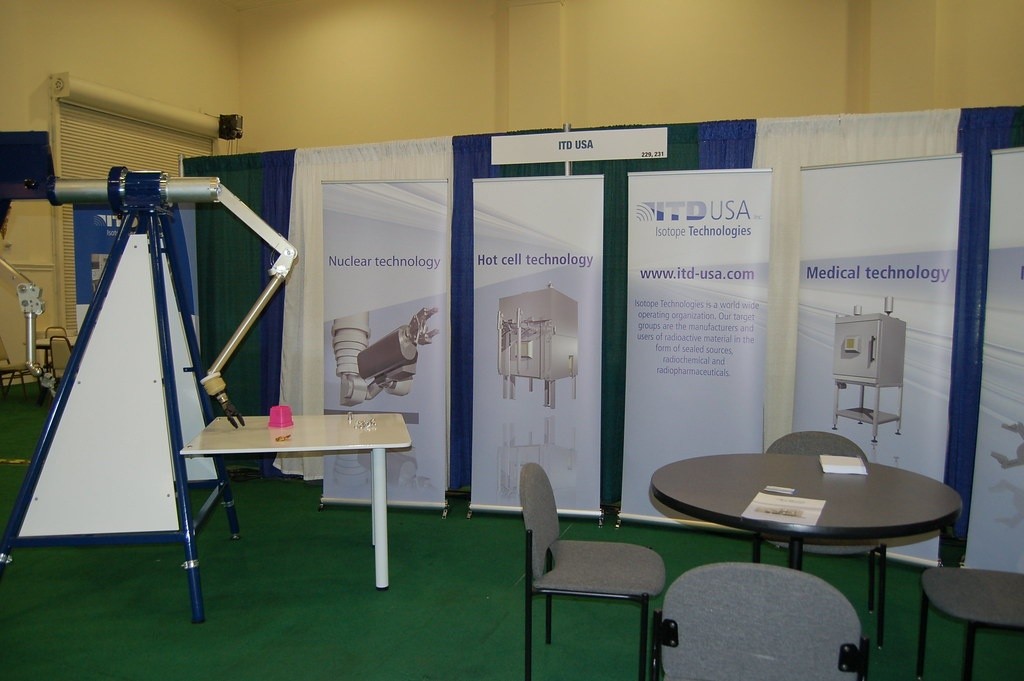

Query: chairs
left=0, top=336, right=32, bottom=404
left=49, top=336, right=73, bottom=410
left=519, top=462, right=667, bottom=680
left=751, top=430, right=877, bottom=614
left=650, top=560, right=873, bottom=681
left=916, top=565, right=1023, bottom=680
left=44, top=326, right=68, bottom=378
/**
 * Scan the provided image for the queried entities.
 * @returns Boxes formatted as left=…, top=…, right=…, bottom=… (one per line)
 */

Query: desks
left=652, top=454, right=963, bottom=580
left=24, top=335, right=78, bottom=378
left=181, top=412, right=411, bottom=591
